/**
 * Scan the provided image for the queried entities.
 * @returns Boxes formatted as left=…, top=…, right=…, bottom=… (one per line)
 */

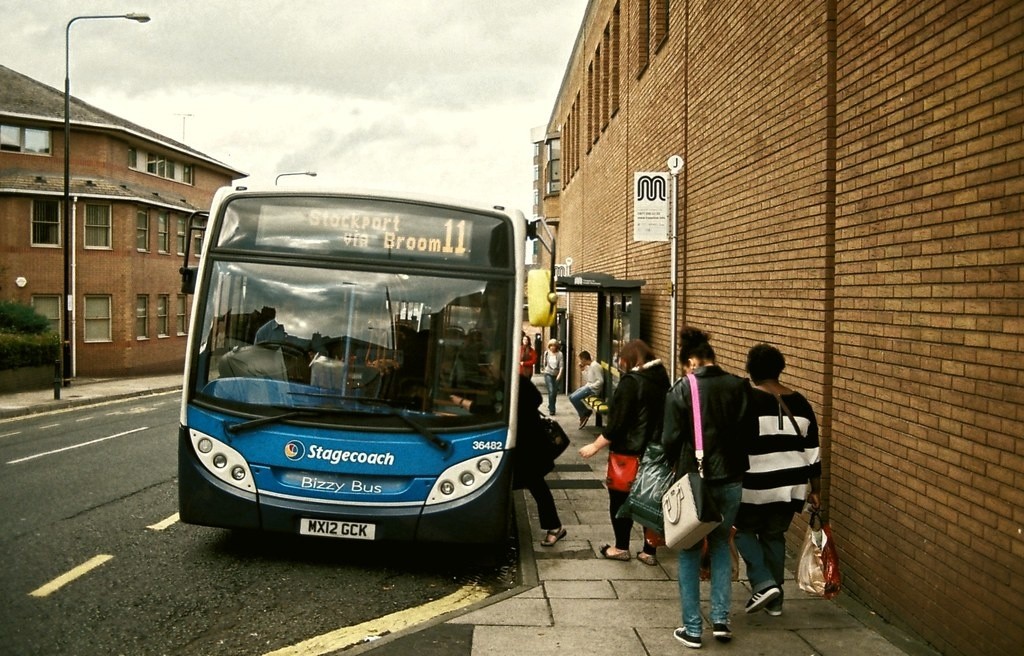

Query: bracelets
left=459, top=397, right=464, bottom=408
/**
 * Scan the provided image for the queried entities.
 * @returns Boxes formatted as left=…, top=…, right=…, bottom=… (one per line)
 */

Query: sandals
left=541, top=527, right=566, bottom=546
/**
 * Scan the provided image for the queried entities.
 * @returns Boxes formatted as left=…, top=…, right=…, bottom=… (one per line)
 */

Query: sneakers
left=579, top=409, right=592, bottom=430
left=712, top=623, right=733, bottom=639
left=673, top=627, right=702, bottom=648
left=745, top=585, right=782, bottom=616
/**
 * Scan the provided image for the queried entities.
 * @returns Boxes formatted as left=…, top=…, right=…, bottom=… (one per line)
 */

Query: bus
left=177, top=185, right=558, bottom=545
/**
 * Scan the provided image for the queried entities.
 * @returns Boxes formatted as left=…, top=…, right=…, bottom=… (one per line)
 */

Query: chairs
left=361, top=319, right=485, bottom=400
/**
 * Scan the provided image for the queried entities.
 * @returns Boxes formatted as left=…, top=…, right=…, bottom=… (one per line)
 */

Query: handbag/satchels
left=797, top=510, right=842, bottom=600
left=615, top=442, right=675, bottom=535
left=605, top=452, right=640, bottom=493
left=659, top=472, right=723, bottom=549
left=536, top=407, right=570, bottom=460
left=700, top=526, right=739, bottom=583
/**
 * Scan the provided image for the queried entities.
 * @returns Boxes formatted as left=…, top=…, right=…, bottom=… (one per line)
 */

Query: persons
left=660, top=327, right=751, bottom=648
left=435, top=327, right=741, bottom=583
left=253, top=290, right=328, bottom=358
left=731, top=343, right=822, bottom=615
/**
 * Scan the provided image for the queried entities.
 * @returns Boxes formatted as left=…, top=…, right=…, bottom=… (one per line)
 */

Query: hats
left=547, top=339, right=559, bottom=348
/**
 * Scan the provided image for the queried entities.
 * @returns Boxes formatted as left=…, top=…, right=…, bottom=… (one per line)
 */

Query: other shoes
left=599, top=544, right=631, bottom=561
left=550, top=411, right=555, bottom=416
left=637, top=550, right=657, bottom=566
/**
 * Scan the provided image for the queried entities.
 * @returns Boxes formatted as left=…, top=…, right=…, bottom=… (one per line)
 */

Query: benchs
left=580, top=361, right=621, bottom=435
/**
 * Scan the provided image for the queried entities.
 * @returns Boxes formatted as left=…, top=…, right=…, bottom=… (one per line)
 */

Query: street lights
left=275, top=171, right=317, bottom=185
left=63, top=13, right=150, bottom=388
